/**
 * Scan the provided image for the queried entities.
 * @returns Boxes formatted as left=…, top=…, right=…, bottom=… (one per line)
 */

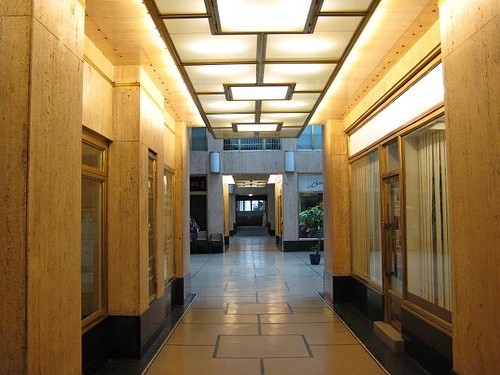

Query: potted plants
left=299, top=203, right=325, bottom=266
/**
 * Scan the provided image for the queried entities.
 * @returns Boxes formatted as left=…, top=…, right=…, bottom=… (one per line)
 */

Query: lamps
left=284, top=150, right=295, bottom=173
left=209, top=150, right=220, bottom=174
